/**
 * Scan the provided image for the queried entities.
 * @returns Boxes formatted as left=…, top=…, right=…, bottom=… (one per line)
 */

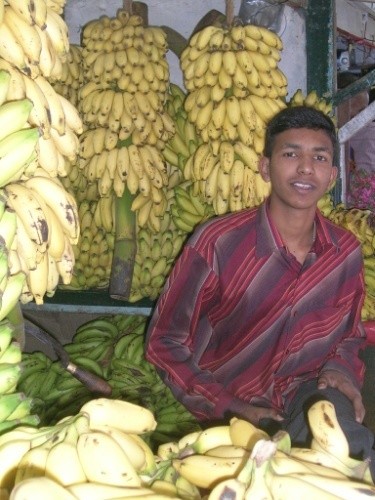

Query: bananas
left=0, top=312, right=375, bottom=499
left=78, top=9, right=179, bottom=302
left=324, top=103, right=333, bottom=115
left=180, top=22, right=288, bottom=260
left=0, top=0, right=84, bottom=436
left=293, top=89, right=303, bottom=106
left=314, top=99, right=327, bottom=111
left=305, top=89, right=317, bottom=107
left=315, top=179, right=375, bottom=323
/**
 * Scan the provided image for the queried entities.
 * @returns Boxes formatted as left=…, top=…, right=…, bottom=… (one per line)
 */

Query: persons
left=143, top=105, right=375, bottom=480
